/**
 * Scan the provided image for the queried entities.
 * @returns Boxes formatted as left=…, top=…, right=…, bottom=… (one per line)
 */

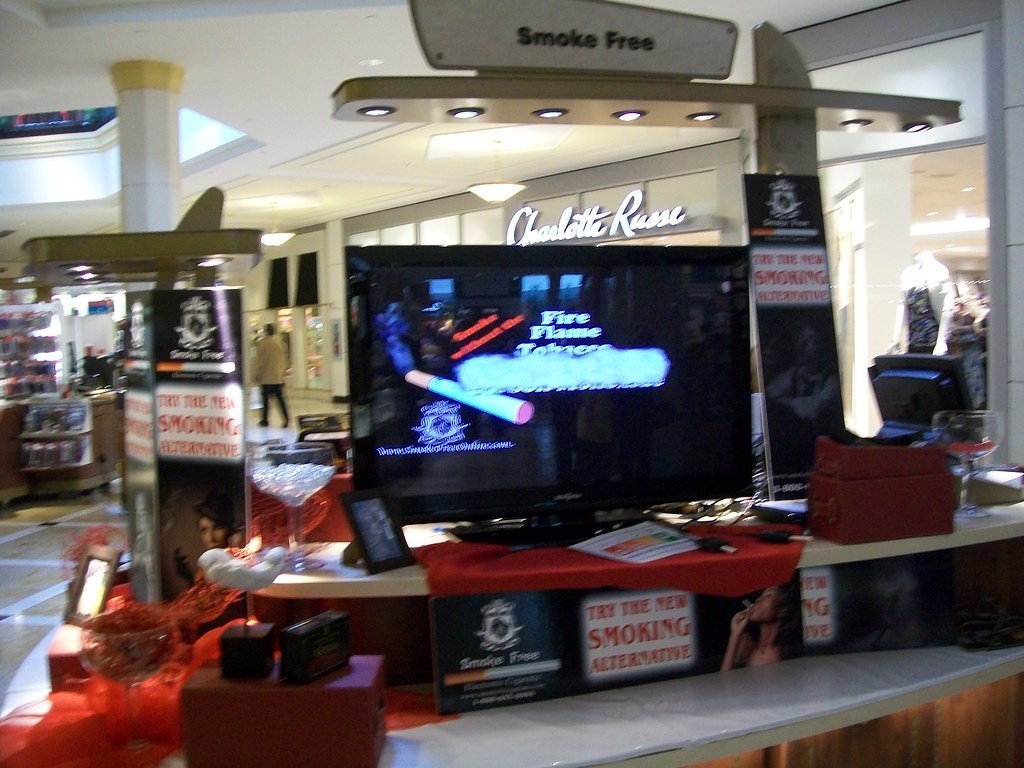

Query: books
left=568, top=519, right=700, bottom=564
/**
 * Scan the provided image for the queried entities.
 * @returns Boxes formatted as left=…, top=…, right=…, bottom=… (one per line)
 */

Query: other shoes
left=260, top=419, right=268, bottom=426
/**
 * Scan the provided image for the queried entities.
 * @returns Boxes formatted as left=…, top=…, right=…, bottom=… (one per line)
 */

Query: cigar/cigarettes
left=125, top=359, right=151, bottom=370
left=404, top=370, right=534, bottom=424
left=444, top=659, right=561, bottom=686
left=156, top=362, right=235, bottom=373
left=742, top=600, right=751, bottom=608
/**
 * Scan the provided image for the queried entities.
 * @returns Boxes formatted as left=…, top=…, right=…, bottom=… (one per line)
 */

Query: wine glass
left=932, top=410, right=1005, bottom=519
left=251, top=441, right=337, bottom=574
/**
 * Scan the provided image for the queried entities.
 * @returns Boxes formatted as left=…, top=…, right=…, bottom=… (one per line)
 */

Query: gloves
left=173, top=547, right=196, bottom=589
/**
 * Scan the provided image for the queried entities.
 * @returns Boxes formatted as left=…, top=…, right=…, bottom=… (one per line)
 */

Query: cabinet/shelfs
left=18, top=395, right=95, bottom=472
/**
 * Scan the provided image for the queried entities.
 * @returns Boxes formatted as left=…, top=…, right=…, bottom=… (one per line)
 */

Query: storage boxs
left=47, top=623, right=86, bottom=691
left=805, top=433, right=956, bottom=545
left=281, top=607, right=349, bottom=685
left=181, top=653, right=391, bottom=768
left=221, top=625, right=273, bottom=678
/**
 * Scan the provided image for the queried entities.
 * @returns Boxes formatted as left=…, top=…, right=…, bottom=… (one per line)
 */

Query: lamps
left=469, top=183, right=528, bottom=205
left=261, top=231, right=295, bottom=247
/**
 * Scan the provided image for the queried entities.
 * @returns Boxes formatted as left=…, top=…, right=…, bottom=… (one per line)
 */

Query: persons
left=74, top=346, right=96, bottom=371
left=252, top=323, right=289, bottom=428
left=172, top=493, right=246, bottom=588
left=719, top=584, right=790, bottom=671
left=889, top=251, right=954, bottom=356
left=952, top=303, right=972, bottom=325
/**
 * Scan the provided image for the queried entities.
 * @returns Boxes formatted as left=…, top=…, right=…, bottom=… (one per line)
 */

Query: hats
left=194, top=494, right=236, bottom=529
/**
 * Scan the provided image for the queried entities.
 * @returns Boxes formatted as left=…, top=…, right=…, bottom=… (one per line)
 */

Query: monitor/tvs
left=868, top=355, right=974, bottom=443
left=344, top=245, right=755, bottom=547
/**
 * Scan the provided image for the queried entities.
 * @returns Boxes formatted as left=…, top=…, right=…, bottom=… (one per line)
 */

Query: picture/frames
left=62, top=542, right=120, bottom=628
left=336, top=486, right=416, bottom=575
